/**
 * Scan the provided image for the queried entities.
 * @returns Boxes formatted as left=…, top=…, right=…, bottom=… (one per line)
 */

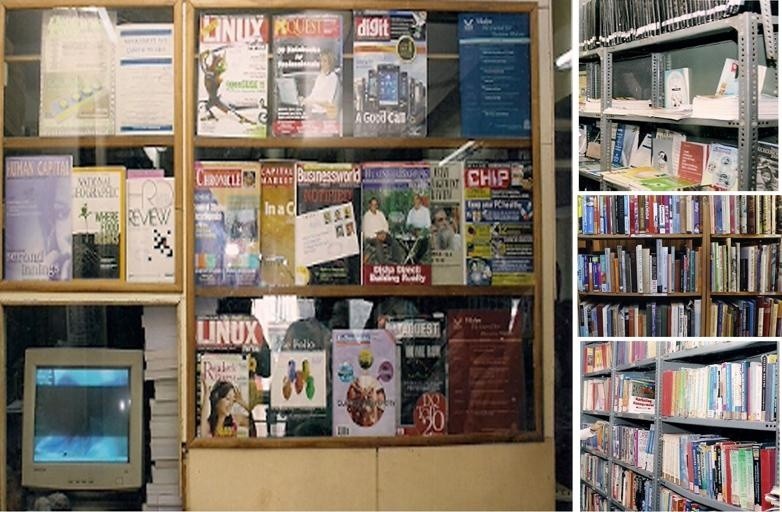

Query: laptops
left=276, top=77, right=304, bottom=107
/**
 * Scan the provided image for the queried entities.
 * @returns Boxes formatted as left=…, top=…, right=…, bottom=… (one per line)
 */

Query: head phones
left=211, top=383, right=233, bottom=401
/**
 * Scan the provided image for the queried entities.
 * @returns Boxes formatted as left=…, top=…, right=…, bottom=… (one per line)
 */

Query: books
left=4, top=6, right=780, bottom=512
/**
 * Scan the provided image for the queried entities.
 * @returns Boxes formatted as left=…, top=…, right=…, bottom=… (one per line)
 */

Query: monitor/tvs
left=20, top=348, right=146, bottom=511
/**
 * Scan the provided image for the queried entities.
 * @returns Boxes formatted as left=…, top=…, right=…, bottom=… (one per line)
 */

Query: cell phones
left=363, top=64, right=417, bottom=114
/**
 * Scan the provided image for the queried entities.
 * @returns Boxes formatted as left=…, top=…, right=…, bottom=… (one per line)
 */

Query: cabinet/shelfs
left=1, top=1, right=184, bottom=511
left=563, top=1, right=778, bottom=512
left=183, top=7, right=562, bottom=512
